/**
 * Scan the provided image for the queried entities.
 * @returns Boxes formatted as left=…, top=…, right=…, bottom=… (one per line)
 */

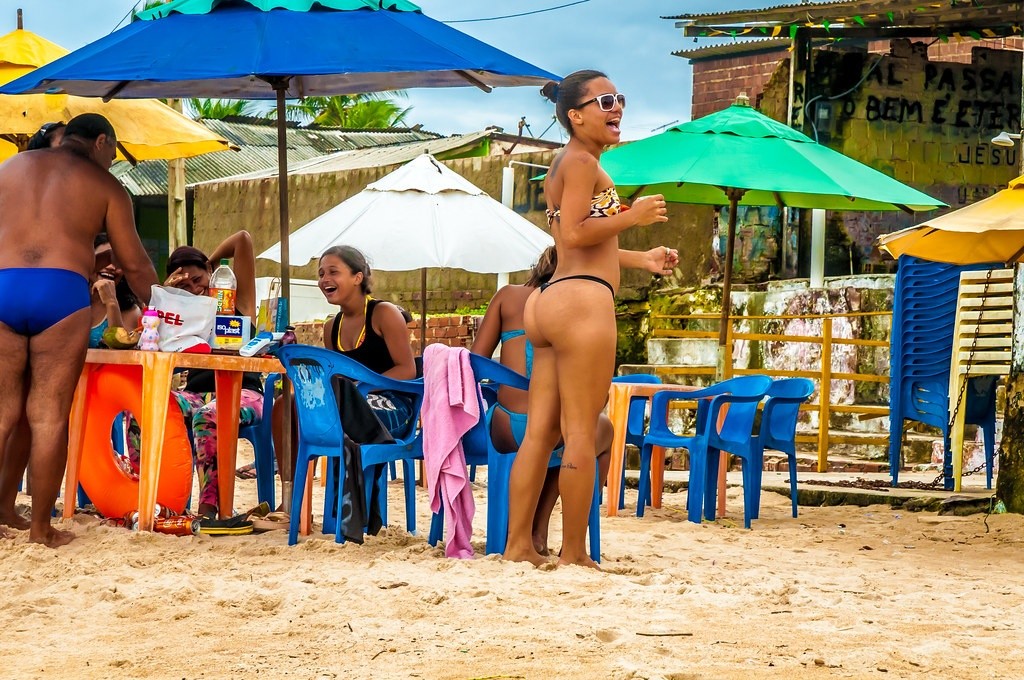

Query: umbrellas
left=525, top=90, right=950, bottom=386
left=255, top=146, right=556, bottom=357
left=877, top=172, right=1024, bottom=265
left=0, top=7, right=241, bottom=159
left=0, top=0, right=562, bottom=324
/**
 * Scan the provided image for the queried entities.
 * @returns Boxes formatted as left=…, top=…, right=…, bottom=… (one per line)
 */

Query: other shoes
left=237, top=468, right=254, bottom=479
left=194, top=513, right=254, bottom=535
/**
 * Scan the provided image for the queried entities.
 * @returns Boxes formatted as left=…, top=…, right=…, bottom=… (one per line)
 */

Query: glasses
left=573, top=94, right=625, bottom=112
left=41, top=123, right=57, bottom=137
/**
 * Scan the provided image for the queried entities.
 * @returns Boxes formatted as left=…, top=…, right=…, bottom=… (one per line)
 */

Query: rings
left=168, top=278, right=174, bottom=285
left=666, top=247, right=670, bottom=255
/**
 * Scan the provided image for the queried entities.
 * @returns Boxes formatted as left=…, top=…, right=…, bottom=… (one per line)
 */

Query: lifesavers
left=69, top=364, right=196, bottom=525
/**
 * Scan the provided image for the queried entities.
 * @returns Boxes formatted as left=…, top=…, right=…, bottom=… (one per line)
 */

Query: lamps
left=991, top=132, right=1023, bottom=147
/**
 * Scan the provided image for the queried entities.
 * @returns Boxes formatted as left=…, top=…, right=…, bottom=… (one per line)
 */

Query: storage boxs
left=210, top=315, right=253, bottom=348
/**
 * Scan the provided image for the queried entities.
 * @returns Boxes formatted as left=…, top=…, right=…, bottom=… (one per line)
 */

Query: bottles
left=138, top=307, right=149, bottom=331
left=279, top=326, right=297, bottom=347
left=208, top=259, right=237, bottom=315
left=140, top=306, right=160, bottom=351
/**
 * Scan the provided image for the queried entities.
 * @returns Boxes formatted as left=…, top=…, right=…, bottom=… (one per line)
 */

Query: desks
left=605, top=381, right=735, bottom=519
left=62, top=345, right=288, bottom=531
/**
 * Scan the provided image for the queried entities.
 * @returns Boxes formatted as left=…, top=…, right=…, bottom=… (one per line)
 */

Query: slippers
left=252, top=511, right=302, bottom=532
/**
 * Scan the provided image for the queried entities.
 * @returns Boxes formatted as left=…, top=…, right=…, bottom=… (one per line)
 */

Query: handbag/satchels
left=149, top=284, right=217, bottom=353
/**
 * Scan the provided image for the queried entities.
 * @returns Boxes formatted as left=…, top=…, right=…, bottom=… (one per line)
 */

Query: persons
left=503, top=70, right=679, bottom=570
left=468, top=245, right=614, bottom=553
left=247, top=245, right=417, bottom=534
left=126, top=229, right=265, bottom=517
left=0, top=113, right=160, bottom=549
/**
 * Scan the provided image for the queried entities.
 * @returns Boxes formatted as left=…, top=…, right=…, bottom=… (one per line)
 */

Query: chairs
left=277, top=345, right=426, bottom=548
left=425, top=338, right=602, bottom=566
left=611, top=373, right=668, bottom=511
left=236, top=372, right=282, bottom=511
left=634, top=372, right=773, bottom=531
left=746, top=377, right=816, bottom=519
left=321, top=355, right=425, bottom=534
left=887, top=252, right=1015, bottom=492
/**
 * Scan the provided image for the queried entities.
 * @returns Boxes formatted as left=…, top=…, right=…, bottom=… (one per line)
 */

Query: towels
left=419, top=344, right=480, bottom=560
